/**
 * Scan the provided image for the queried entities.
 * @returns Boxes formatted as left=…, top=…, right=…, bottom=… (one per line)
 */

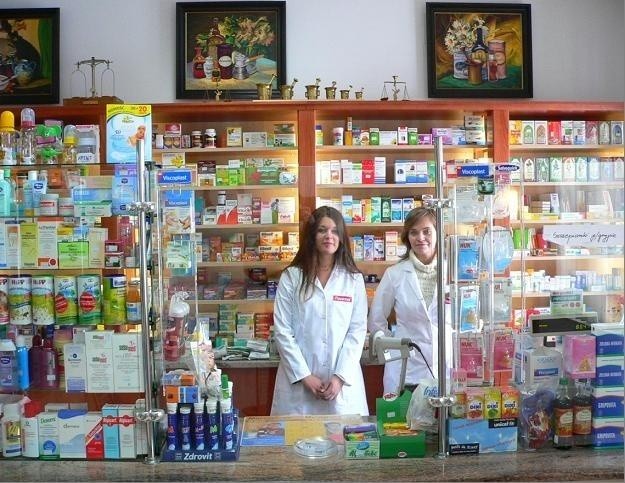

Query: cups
left=254, top=82, right=364, bottom=100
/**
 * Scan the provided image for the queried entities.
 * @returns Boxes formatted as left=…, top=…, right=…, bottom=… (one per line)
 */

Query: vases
left=452, top=53, right=469, bottom=80
left=232, top=46, right=259, bottom=74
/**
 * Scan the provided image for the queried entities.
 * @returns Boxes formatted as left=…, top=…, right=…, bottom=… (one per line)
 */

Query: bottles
left=1, top=106, right=97, bottom=163
left=553, top=375, right=593, bottom=452
left=0, top=333, right=61, bottom=389
left=0, top=397, right=42, bottom=459
left=316, top=125, right=344, bottom=146
left=217, top=190, right=227, bottom=205
left=125, top=280, right=141, bottom=325
left=191, top=128, right=217, bottom=149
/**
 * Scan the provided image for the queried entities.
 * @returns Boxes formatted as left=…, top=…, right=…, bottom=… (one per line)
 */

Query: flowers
left=217, top=14, right=275, bottom=55
left=444, top=14, right=490, bottom=55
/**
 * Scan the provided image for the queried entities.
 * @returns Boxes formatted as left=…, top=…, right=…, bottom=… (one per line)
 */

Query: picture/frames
left=174, top=2, right=287, bottom=100
left=425, top=2, right=534, bottom=97
left=0, top=9, right=61, bottom=105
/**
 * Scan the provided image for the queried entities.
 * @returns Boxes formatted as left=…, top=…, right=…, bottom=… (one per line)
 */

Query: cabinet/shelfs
left=0, top=96, right=625, bottom=416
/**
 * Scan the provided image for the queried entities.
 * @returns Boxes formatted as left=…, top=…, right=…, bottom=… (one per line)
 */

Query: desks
left=436, top=64, right=520, bottom=89
left=12, top=78, right=51, bottom=94
left=185, top=57, right=276, bottom=90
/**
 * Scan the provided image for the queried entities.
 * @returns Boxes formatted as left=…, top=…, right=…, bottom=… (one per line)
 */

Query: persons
left=271, top=204, right=371, bottom=416
left=365, top=206, right=454, bottom=395
